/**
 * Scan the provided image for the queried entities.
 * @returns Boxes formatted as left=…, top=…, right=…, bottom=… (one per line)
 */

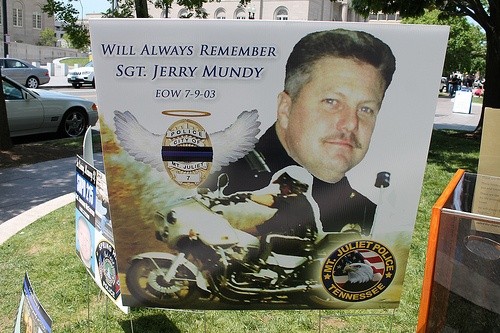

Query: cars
left=0, top=58, right=50, bottom=90
left=0, top=75, right=98, bottom=143
left=67, top=61, right=97, bottom=87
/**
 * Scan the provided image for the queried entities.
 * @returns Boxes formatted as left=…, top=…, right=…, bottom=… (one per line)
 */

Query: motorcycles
left=128, top=175, right=361, bottom=308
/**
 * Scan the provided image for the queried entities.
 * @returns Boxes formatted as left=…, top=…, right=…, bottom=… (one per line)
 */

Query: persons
left=448, top=73, right=475, bottom=97
left=75, top=216, right=95, bottom=279
left=198, top=28, right=396, bottom=236
